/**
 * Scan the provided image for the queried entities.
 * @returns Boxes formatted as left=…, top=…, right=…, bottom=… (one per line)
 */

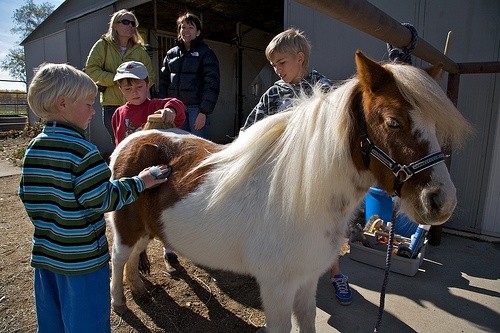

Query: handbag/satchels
left=82, top=66, right=107, bottom=93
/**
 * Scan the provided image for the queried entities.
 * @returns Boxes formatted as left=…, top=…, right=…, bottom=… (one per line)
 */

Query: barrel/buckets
left=365, top=186, right=419, bottom=239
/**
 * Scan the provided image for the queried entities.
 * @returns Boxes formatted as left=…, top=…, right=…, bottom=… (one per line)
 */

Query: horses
left=107, top=50, right=474, bottom=333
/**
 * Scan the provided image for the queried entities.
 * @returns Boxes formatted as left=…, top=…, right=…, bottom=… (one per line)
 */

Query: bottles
left=408, top=225, right=432, bottom=258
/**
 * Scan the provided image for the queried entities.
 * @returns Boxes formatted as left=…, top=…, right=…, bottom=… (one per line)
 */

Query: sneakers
left=163, top=252, right=181, bottom=275
left=331, top=273, right=353, bottom=305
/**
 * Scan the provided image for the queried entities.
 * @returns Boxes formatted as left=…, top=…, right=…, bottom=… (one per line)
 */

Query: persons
left=239, top=29, right=356, bottom=306
left=110, top=61, right=186, bottom=275
left=86, top=8, right=154, bottom=149
left=18, top=62, right=168, bottom=333
left=158, top=13, right=220, bottom=140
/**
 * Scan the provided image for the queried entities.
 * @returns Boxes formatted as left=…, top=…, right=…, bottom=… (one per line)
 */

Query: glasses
left=115, top=19, right=135, bottom=27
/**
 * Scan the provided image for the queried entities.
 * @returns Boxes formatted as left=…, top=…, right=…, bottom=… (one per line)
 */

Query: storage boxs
left=348, top=227, right=427, bottom=277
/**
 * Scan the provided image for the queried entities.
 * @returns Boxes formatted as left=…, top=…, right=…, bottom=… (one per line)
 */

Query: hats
left=113, top=60, right=148, bottom=82
